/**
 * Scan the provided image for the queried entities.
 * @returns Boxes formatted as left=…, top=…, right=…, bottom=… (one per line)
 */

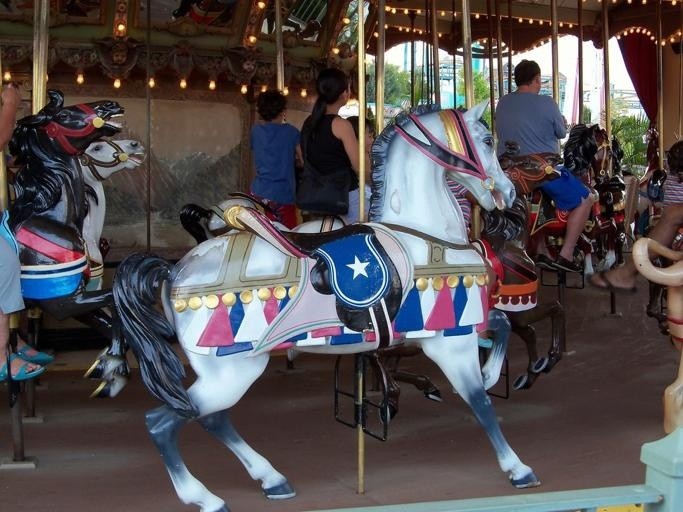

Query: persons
left=494, top=60, right=593, bottom=274
left=295, top=68, right=365, bottom=220
left=1, top=84, right=53, bottom=377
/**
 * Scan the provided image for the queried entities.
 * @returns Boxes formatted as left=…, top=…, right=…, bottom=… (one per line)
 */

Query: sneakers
left=552, top=255, right=581, bottom=272
left=535, top=253, right=558, bottom=272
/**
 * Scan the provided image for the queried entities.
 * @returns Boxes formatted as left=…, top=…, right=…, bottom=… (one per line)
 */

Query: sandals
left=16, top=344, right=54, bottom=365
left=0, top=353, right=45, bottom=383
left=585, top=271, right=637, bottom=296
